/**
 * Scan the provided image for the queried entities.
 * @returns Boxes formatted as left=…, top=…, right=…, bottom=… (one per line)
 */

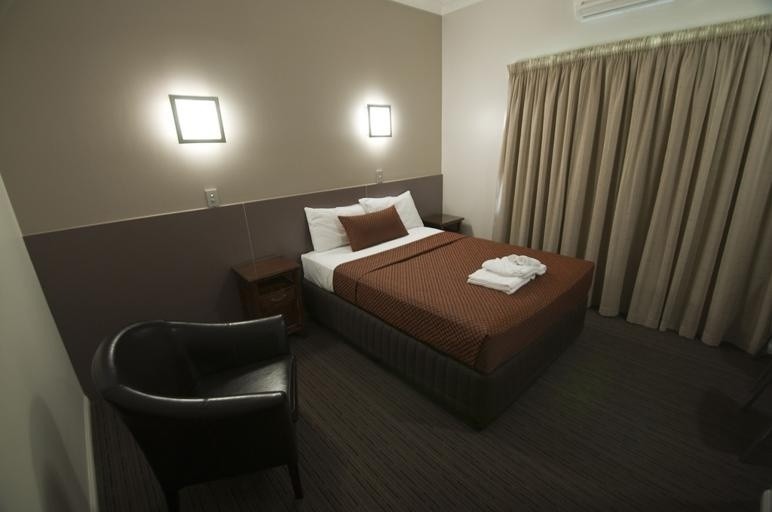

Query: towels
left=466, top=253, right=547, bottom=296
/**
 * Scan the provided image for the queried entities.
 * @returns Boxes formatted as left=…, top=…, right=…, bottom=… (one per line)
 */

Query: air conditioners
left=574, top=0, right=675, bottom=24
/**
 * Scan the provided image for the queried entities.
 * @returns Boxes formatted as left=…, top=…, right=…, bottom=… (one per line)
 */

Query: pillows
left=304, top=204, right=366, bottom=254
left=358, top=190, right=425, bottom=230
left=337, top=204, right=410, bottom=252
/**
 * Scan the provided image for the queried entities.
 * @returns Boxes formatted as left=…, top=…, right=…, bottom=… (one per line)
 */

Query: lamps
left=367, top=104, right=392, bottom=137
left=168, top=95, right=227, bottom=144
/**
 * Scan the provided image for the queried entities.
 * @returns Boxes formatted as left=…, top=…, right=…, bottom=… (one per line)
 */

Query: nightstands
left=423, top=213, right=464, bottom=232
left=231, top=253, right=304, bottom=320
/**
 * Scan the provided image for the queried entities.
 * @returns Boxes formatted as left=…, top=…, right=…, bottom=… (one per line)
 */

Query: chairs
left=90, top=313, right=304, bottom=512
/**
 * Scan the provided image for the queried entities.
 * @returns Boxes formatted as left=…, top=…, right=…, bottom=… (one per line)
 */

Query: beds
left=301, top=227, right=595, bottom=431
